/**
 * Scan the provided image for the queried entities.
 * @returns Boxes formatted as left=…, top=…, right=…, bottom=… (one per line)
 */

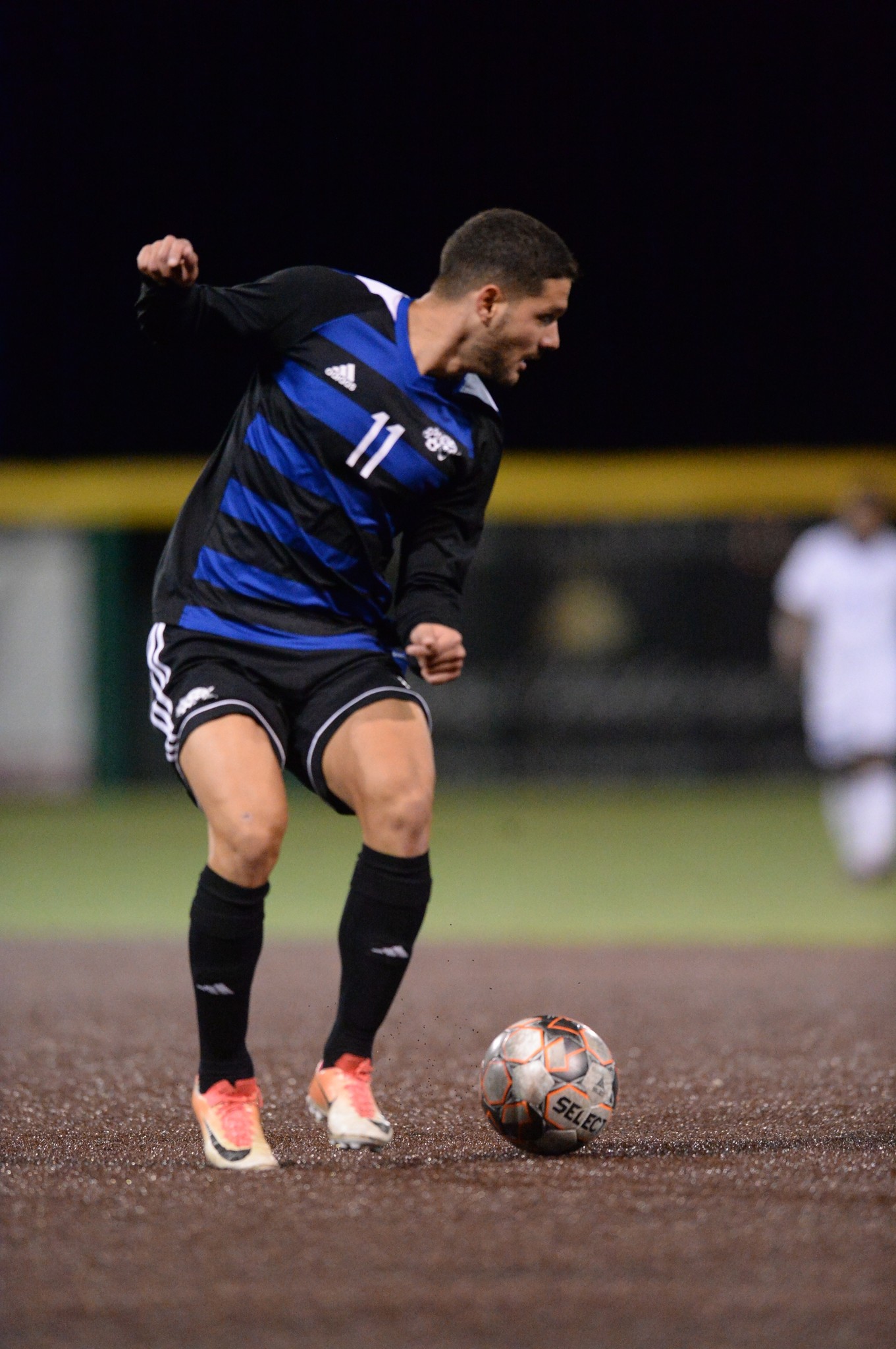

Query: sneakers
left=191, top=1072, right=280, bottom=1173
left=305, top=1053, right=395, bottom=1149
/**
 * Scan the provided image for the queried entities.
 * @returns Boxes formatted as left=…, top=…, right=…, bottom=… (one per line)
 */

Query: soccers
left=477, top=1013, right=622, bottom=1159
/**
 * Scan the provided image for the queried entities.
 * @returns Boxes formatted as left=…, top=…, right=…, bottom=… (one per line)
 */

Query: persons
left=122, top=196, right=580, bottom=1179
left=767, top=475, right=895, bottom=879
